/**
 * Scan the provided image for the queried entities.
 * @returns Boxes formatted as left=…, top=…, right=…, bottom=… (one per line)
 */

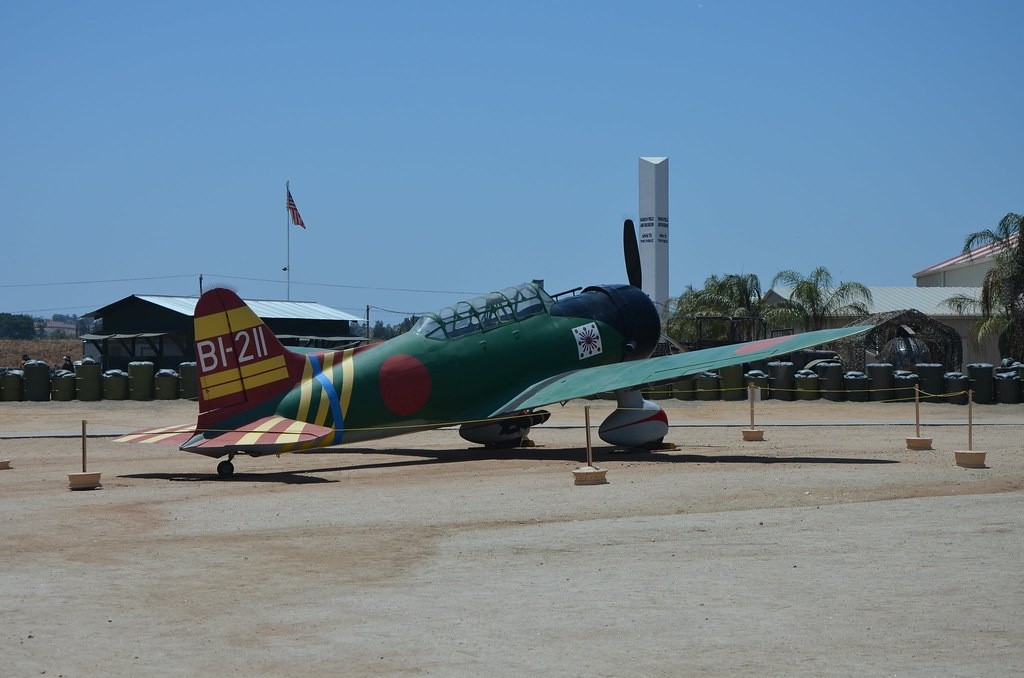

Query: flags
left=286, top=184, right=306, bottom=229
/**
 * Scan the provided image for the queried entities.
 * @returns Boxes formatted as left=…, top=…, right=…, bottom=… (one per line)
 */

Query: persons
left=62, top=355, right=74, bottom=373
left=19, top=354, right=31, bottom=368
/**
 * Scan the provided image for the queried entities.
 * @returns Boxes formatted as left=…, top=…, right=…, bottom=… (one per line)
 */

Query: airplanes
left=105, top=216, right=880, bottom=479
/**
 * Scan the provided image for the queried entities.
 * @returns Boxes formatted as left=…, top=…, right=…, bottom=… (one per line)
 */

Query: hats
left=62, top=355, right=71, bottom=361
